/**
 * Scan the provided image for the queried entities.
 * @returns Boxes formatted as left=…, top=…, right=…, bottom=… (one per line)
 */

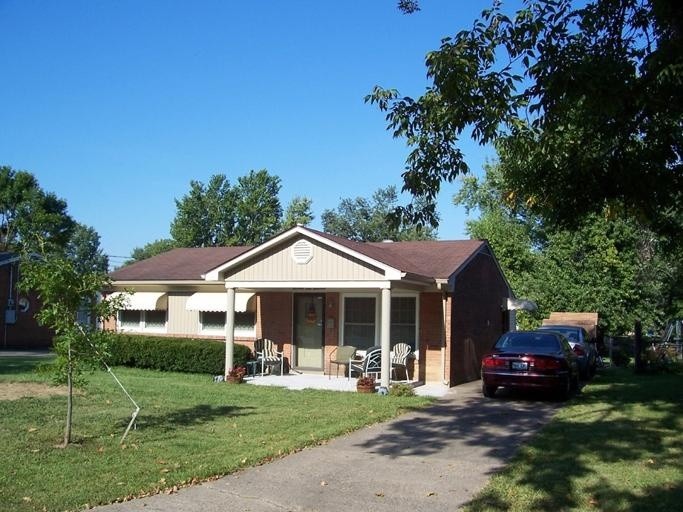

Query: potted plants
left=356, top=377, right=377, bottom=393
left=226, top=367, right=246, bottom=383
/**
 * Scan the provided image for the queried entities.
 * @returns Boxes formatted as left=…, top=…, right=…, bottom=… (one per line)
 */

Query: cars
left=479, top=324, right=596, bottom=401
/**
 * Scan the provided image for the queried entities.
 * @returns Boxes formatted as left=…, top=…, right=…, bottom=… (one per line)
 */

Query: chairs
left=254, top=338, right=284, bottom=377
left=328, top=343, right=413, bottom=383
